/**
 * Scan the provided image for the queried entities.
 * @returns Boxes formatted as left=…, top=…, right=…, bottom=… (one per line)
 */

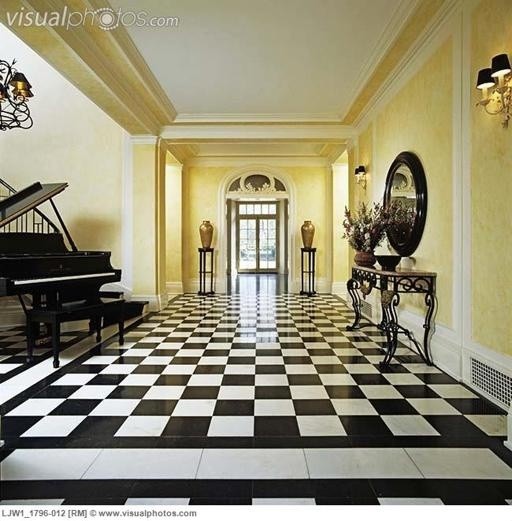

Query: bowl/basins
left=375, top=255, right=402, bottom=272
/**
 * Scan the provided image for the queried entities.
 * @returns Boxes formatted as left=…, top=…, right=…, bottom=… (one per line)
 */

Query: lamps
left=0, top=58, right=34, bottom=131
left=476, top=54, right=512, bottom=129
left=354, top=165, right=367, bottom=191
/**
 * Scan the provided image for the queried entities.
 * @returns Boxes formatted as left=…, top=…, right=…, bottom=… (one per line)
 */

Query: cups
left=400, top=257, right=410, bottom=270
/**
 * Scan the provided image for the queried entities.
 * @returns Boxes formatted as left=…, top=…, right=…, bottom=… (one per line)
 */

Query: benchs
left=25, top=298, right=126, bottom=368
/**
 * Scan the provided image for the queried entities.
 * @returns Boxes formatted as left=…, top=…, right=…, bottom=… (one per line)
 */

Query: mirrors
left=383, top=151, right=427, bottom=257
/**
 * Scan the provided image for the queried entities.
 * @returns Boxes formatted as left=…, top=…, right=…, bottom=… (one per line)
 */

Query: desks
left=346, top=266, right=437, bottom=366
left=198, top=248, right=215, bottom=295
left=300, top=248, right=316, bottom=297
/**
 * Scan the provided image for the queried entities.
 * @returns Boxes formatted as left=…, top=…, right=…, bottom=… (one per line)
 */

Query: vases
left=301, top=221, right=315, bottom=248
left=354, top=251, right=376, bottom=267
left=200, top=221, right=213, bottom=248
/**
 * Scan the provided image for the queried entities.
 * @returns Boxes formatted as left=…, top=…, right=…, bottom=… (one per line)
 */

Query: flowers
left=341, top=199, right=405, bottom=252
left=391, top=206, right=417, bottom=244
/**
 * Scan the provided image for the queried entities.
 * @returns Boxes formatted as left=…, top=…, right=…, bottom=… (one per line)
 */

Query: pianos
left=0, top=181, right=149, bottom=368
